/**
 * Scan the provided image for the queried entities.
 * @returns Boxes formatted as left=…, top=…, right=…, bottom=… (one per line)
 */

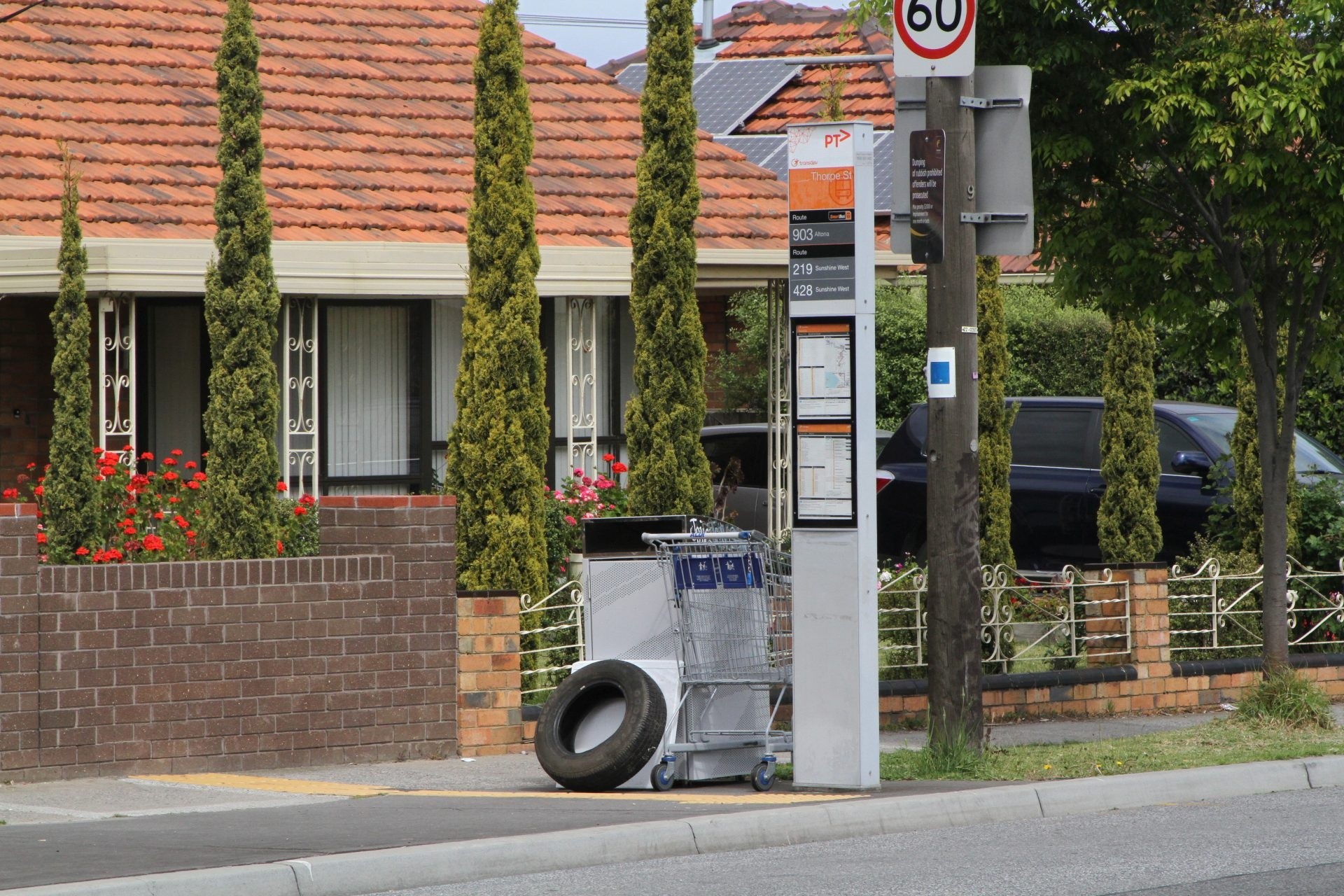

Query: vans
left=877, top=394, right=1343, bottom=570
left=693, top=421, right=896, bottom=541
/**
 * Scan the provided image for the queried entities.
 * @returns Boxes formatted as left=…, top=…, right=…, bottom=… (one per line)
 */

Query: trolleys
left=643, top=527, right=793, bottom=793
